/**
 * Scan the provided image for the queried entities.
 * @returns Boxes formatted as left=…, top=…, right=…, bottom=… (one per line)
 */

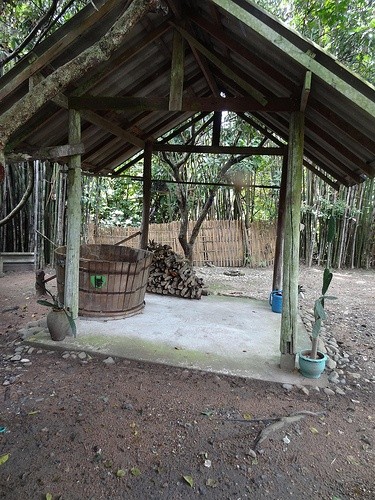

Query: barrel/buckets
left=269, top=290, right=284, bottom=314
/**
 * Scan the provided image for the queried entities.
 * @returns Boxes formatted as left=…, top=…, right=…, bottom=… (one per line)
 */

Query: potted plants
left=297, top=268, right=334, bottom=379
left=34, top=281, right=82, bottom=341
